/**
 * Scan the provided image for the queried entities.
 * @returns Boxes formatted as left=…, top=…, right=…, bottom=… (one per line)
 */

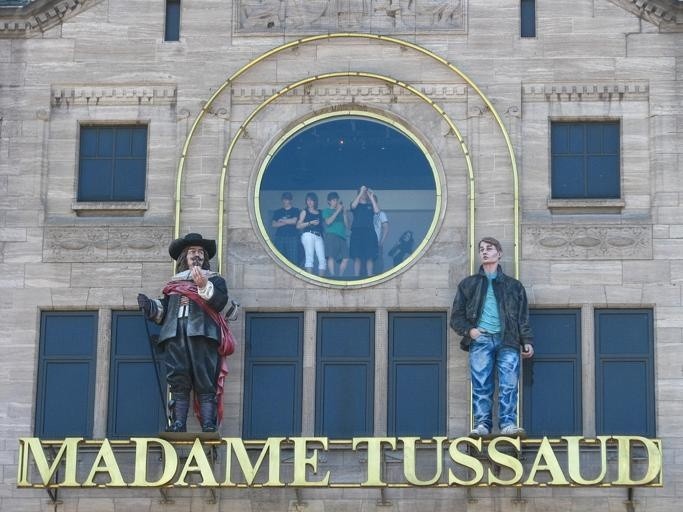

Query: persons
left=138, top=233, right=235, bottom=432
left=272, top=186, right=413, bottom=278
left=450, top=237, right=534, bottom=438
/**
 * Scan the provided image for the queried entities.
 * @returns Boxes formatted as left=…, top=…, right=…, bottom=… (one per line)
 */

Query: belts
left=305, top=230, right=320, bottom=237
